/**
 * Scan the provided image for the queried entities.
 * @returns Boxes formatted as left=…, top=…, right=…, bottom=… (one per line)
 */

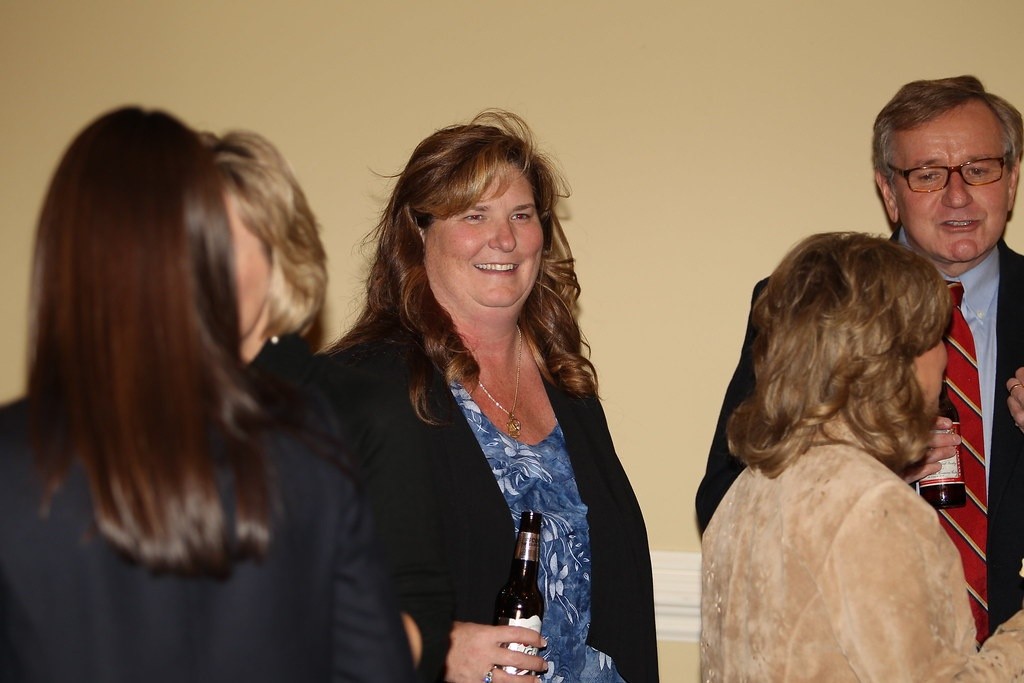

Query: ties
left=937, top=280, right=991, bottom=645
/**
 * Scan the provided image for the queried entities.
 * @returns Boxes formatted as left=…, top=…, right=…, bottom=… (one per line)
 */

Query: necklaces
left=477, top=326, right=522, bottom=437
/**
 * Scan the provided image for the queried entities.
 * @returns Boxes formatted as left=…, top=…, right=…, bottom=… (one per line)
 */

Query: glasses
left=886, top=150, right=1013, bottom=193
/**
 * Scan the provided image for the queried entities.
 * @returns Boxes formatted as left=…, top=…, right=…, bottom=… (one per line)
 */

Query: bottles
left=491, top=511, right=544, bottom=676
left=916, top=373, right=966, bottom=508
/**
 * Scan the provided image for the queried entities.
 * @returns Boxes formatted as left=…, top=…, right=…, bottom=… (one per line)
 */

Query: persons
left=697, top=231, right=1024, bottom=683
left=696, top=74, right=1024, bottom=646
left=298, top=124, right=663, bottom=683
left=0, top=106, right=417, bottom=683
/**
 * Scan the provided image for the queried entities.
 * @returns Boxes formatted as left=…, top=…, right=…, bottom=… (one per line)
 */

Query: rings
left=485, top=668, right=494, bottom=683
